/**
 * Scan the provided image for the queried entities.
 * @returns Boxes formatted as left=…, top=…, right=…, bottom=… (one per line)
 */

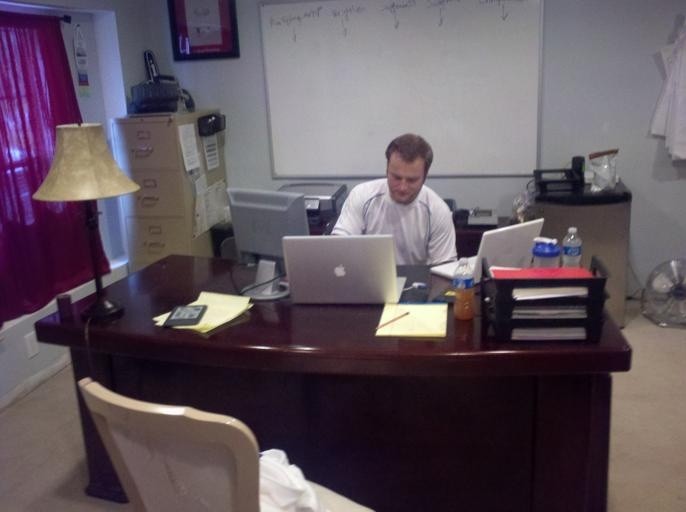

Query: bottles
left=451, top=256, right=475, bottom=320
left=561, top=226, right=583, bottom=269
left=533, top=236, right=561, bottom=269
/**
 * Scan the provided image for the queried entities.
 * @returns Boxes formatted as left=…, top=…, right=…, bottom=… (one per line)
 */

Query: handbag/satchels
left=128, top=74, right=195, bottom=114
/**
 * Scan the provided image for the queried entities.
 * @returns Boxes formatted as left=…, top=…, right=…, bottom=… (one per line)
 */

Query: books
left=490, top=262, right=594, bottom=344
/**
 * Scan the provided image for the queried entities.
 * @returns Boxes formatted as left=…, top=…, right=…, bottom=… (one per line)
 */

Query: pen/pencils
left=372, top=311, right=410, bottom=332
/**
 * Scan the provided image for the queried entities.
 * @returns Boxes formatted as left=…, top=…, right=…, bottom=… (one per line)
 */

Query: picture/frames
left=167, top=0, right=241, bottom=64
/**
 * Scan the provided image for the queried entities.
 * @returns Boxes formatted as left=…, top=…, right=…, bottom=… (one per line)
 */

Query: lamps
left=31, top=122, right=141, bottom=320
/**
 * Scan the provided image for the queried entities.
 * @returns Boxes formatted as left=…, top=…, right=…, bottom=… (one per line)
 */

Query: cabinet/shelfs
left=520, top=183, right=633, bottom=331
left=113, top=106, right=230, bottom=276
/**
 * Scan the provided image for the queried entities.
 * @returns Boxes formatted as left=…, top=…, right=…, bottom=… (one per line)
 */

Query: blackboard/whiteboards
left=258, top=0, right=547, bottom=180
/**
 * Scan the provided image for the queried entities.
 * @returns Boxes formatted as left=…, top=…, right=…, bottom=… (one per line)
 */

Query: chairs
left=78, top=378, right=376, bottom=512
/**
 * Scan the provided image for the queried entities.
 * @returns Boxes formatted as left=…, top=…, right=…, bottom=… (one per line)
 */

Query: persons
left=331, top=131, right=460, bottom=264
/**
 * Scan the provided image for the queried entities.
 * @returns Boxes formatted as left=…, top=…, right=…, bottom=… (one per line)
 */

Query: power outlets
left=23, top=332, right=40, bottom=360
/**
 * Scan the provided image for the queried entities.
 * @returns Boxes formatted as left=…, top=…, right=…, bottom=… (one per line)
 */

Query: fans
left=642, top=258, right=686, bottom=330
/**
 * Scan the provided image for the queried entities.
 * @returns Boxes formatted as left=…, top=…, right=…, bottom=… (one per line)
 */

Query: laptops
left=282, top=234, right=407, bottom=304
left=430, top=218, right=545, bottom=286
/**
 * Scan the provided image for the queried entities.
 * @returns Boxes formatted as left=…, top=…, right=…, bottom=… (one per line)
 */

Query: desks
left=33, top=253, right=634, bottom=512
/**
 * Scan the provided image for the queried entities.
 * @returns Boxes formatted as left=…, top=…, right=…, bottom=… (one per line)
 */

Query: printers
left=277, top=184, right=347, bottom=226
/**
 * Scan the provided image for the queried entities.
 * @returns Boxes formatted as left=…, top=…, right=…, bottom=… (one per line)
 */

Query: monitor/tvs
left=226, top=188, right=310, bottom=300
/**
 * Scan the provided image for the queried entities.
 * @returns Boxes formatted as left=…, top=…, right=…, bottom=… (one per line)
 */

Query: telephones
left=454, top=208, right=498, bottom=227
